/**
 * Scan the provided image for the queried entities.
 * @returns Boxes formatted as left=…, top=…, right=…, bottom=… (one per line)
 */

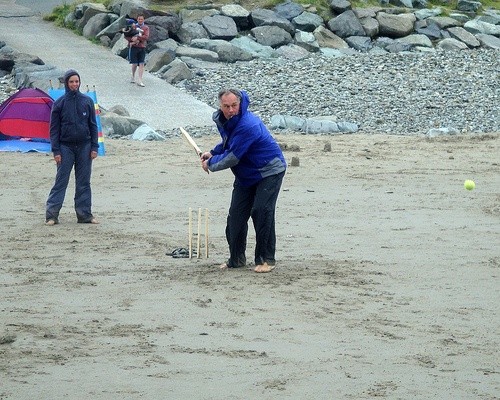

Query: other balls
left=464, top=179, right=476, bottom=190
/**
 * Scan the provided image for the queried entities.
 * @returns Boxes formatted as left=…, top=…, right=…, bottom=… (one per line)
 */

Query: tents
left=0, top=87, right=56, bottom=143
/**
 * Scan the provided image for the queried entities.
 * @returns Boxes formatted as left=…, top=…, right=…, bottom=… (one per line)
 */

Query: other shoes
left=131, top=75, right=136, bottom=83
left=137, top=80, right=146, bottom=87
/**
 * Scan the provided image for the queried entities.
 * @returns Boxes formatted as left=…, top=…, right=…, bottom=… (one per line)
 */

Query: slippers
left=166, top=248, right=197, bottom=258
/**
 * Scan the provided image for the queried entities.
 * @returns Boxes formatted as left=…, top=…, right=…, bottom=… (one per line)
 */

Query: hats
left=126, top=19, right=136, bottom=25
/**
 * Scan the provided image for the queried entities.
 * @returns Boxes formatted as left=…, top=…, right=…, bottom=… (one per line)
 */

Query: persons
left=124, top=14, right=149, bottom=87
left=200, top=88, right=287, bottom=273
left=45, top=70, right=101, bottom=226
